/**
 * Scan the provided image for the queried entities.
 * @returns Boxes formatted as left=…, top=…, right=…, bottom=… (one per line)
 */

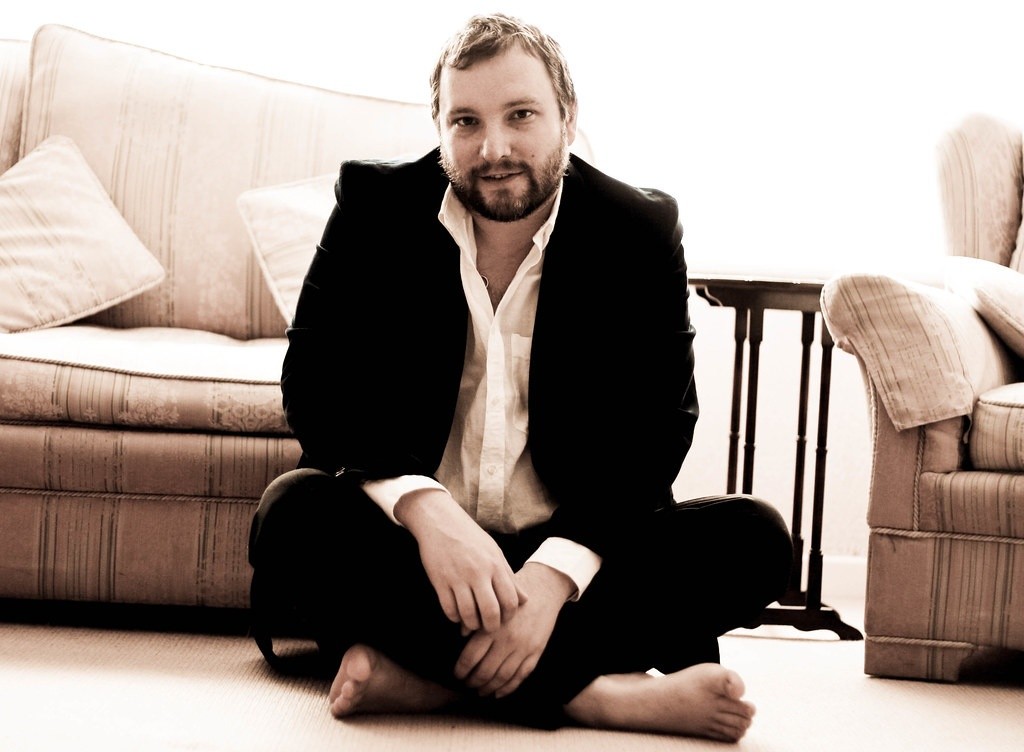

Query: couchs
left=1, top=23, right=443, bottom=606
left=821, top=110, right=1024, bottom=682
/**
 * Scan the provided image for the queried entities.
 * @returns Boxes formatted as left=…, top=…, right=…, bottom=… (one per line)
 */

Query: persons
left=247, top=12, right=796, bottom=746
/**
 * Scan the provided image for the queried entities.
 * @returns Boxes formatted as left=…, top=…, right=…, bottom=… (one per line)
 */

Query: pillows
left=943, top=255, right=1024, bottom=356
left=0, top=134, right=168, bottom=333
left=233, top=176, right=339, bottom=330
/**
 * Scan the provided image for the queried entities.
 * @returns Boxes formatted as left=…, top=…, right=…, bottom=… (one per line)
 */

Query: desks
left=687, top=277, right=866, bottom=639
